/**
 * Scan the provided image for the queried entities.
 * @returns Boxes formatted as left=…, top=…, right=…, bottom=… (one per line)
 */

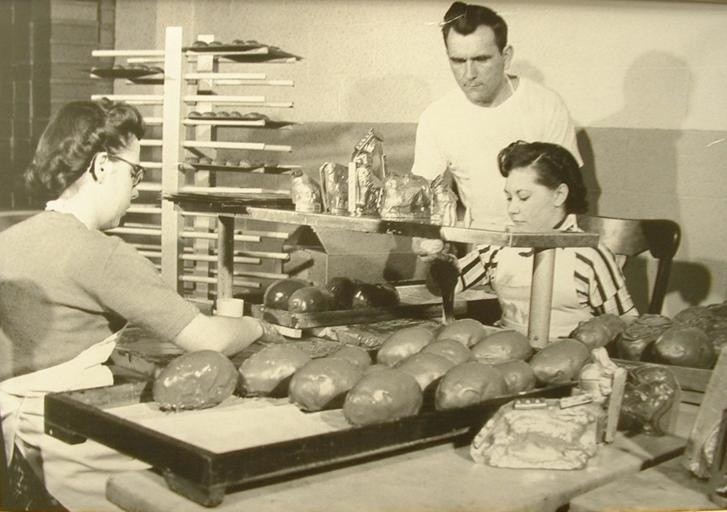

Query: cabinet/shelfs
left=179, top=197, right=601, bottom=346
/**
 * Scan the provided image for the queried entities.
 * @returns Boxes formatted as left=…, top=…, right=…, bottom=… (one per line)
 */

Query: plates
left=44, top=378, right=581, bottom=509
left=260, top=280, right=501, bottom=328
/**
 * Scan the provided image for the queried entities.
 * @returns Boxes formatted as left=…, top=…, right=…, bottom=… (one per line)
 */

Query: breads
left=112, top=64, right=124, bottom=71
left=134, top=65, right=149, bottom=70
left=264, top=276, right=307, bottom=312
left=244, top=113, right=265, bottom=118
left=375, top=325, right=433, bottom=364
left=200, top=111, right=216, bottom=118
left=287, top=285, right=338, bottom=314
left=215, top=112, right=229, bottom=118
left=208, top=41, right=222, bottom=48
left=652, top=327, right=716, bottom=372
left=185, top=156, right=199, bottom=162
left=494, top=359, right=537, bottom=397
left=343, top=365, right=423, bottom=428
left=239, top=159, right=255, bottom=169
left=420, top=340, right=473, bottom=367
left=288, top=356, right=361, bottom=414
left=233, top=38, right=245, bottom=48
left=240, top=344, right=312, bottom=399
left=394, top=353, right=448, bottom=409
left=152, top=348, right=238, bottom=413
left=225, top=159, right=240, bottom=167
left=192, top=39, right=208, bottom=48
left=199, top=156, right=212, bottom=164
left=617, top=311, right=674, bottom=362
left=435, top=361, right=506, bottom=414
left=571, top=314, right=626, bottom=355
left=213, top=158, right=226, bottom=166
left=323, top=276, right=357, bottom=309
left=469, top=330, right=534, bottom=365
left=673, top=306, right=726, bottom=342
left=186, top=109, right=200, bottom=118
left=335, top=343, right=372, bottom=370
left=246, top=40, right=258, bottom=47
left=530, top=339, right=593, bottom=385
left=228, top=111, right=242, bottom=119
left=435, top=317, right=487, bottom=348
left=149, top=67, right=164, bottom=73
left=126, top=63, right=133, bottom=69
left=353, top=282, right=399, bottom=313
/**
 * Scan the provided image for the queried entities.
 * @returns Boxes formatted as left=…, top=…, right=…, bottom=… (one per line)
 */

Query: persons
left=410, top=1, right=585, bottom=231
left=454, top=140, right=637, bottom=340
left=0, top=96, right=284, bottom=511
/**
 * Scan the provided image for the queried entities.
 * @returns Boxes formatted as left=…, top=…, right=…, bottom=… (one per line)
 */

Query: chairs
left=572, top=212, right=682, bottom=316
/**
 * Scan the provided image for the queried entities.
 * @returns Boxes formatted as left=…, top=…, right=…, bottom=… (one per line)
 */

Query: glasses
left=108, top=153, right=145, bottom=187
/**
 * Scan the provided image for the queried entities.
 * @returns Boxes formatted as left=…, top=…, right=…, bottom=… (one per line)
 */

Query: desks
left=105, top=342, right=694, bottom=508
